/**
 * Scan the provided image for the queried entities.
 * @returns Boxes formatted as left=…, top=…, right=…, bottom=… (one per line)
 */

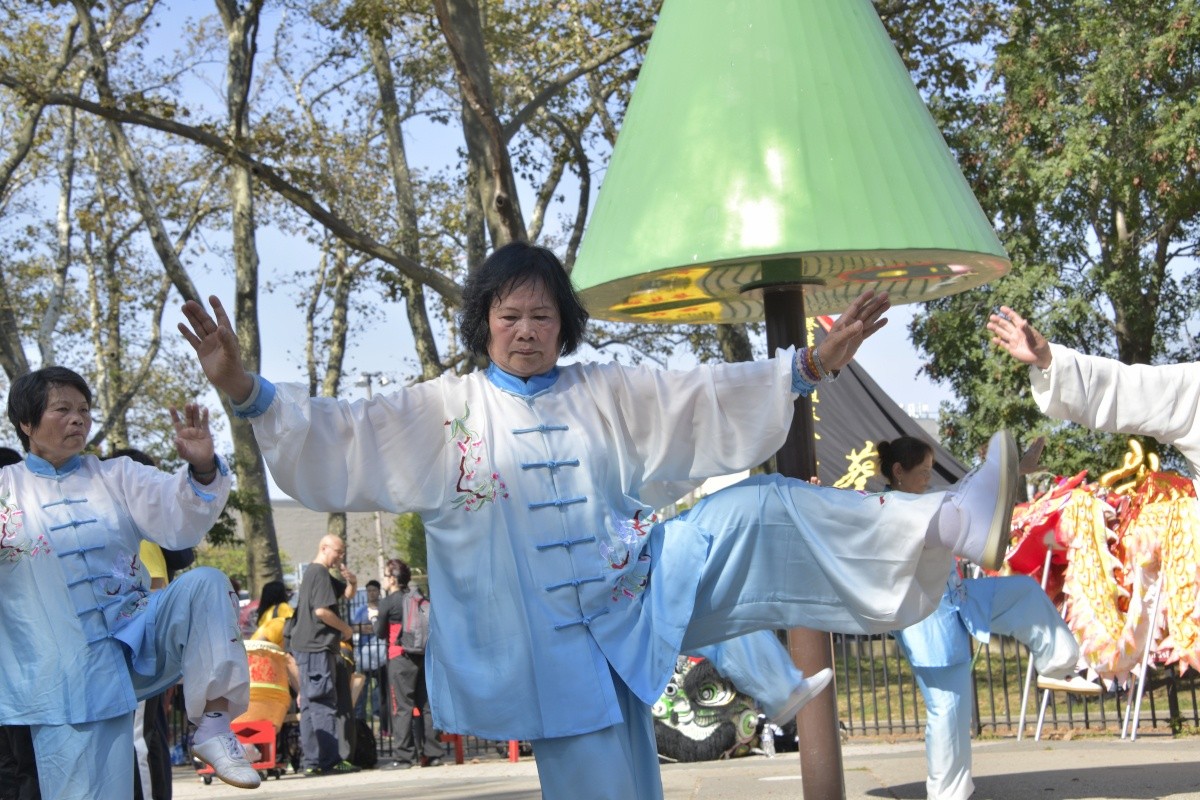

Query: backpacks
left=394, top=588, right=430, bottom=655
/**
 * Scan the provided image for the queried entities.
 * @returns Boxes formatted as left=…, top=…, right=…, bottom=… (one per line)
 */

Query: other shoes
left=379, top=759, right=411, bottom=769
left=329, top=760, right=361, bottom=772
left=767, top=667, right=836, bottom=728
left=420, top=753, right=445, bottom=766
left=303, top=763, right=328, bottom=775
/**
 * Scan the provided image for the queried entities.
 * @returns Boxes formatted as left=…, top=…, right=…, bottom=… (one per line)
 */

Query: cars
left=237, top=574, right=380, bottom=647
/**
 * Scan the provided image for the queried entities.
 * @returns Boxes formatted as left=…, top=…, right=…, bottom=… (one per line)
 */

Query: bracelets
left=796, top=346, right=841, bottom=385
left=229, top=373, right=260, bottom=411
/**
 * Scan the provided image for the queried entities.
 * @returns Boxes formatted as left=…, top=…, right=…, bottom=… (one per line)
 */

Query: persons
left=0, top=367, right=263, bottom=800
left=986, top=305, right=1200, bottom=469
left=250, top=534, right=455, bottom=778
left=804, top=435, right=1103, bottom=800
left=626, top=475, right=833, bottom=728
left=178, top=241, right=1017, bottom=800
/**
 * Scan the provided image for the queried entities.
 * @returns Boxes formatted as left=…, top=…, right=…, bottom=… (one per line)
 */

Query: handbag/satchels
left=353, top=630, right=388, bottom=670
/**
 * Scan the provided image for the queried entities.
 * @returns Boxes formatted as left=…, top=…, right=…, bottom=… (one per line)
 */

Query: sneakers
left=192, top=729, right=260, bottom=789
left=1035, top=667, right=1103, bottom=694
left=950, top=427, right=1019, bottom=569
